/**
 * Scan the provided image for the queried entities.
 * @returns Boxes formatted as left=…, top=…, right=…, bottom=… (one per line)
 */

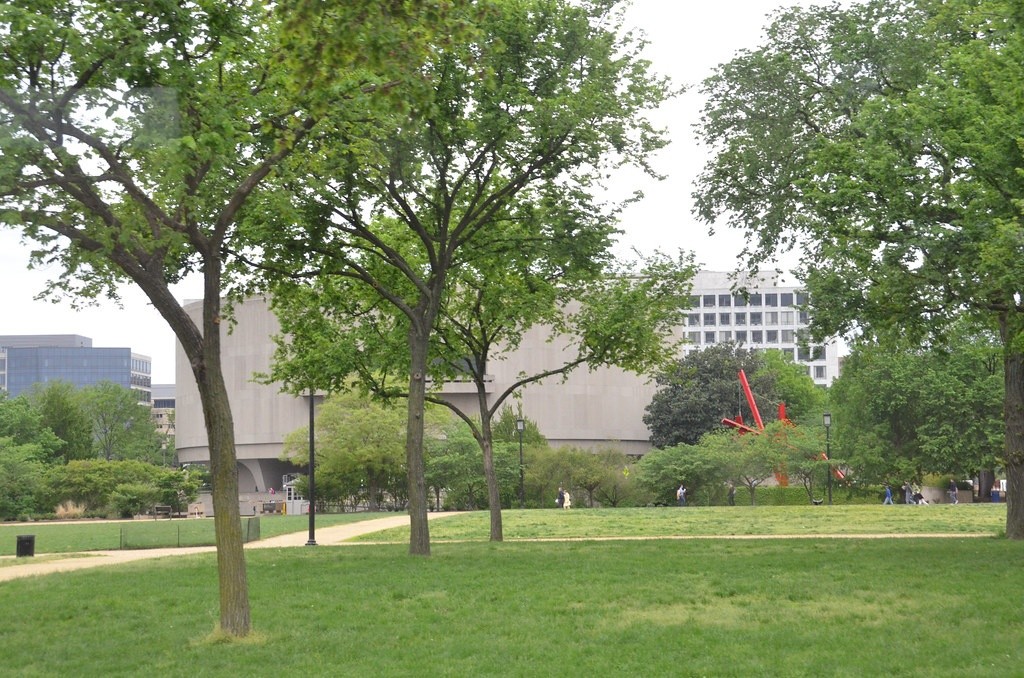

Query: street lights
left=516, top=419, right=524, bottom=508
left=823, top=413, right=832, bottom=504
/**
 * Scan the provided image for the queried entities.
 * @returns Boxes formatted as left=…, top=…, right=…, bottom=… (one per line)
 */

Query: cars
left=254, top=500, right=283, bottom=514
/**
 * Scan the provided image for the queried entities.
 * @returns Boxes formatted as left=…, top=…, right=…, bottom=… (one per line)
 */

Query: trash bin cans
left=16, top=534, right=35, bottom=557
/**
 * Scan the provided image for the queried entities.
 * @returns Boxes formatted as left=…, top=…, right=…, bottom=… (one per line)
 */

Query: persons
left=678, top=484, right=686, bottom=507
left=903, top=480, right=917, bottom=504
left=946, top=479, right=959, bottom=504
left=726, top=483, right=736, bottom=506
left=558, top=487, right=571, bottom=509
left=883, top=486, right=894, bottom=505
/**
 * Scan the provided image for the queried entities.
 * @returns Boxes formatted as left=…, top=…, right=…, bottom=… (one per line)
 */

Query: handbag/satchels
left=555, top=499, right=559, bottom=503
left=914, top=494, right=923, bottom=499
